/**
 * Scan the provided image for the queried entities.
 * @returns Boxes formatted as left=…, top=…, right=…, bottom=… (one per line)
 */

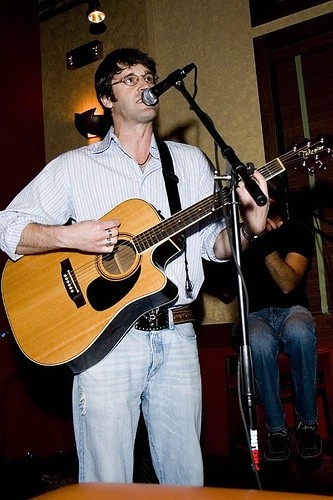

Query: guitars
left=1, top=136, right=333, bottom=376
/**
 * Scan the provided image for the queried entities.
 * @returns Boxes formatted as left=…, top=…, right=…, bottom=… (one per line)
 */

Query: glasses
left=108, top=72, right=159, bottom=87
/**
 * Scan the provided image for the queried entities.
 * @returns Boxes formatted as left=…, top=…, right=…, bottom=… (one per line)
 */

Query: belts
left=136, top=305, right=195, bottom=332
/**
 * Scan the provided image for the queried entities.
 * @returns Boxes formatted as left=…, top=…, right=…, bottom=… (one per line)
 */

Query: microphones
left=142, top=62, right=196, bottom=106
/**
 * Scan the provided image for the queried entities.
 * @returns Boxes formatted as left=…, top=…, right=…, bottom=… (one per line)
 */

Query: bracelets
left=242, top=221, right=265, bottom=243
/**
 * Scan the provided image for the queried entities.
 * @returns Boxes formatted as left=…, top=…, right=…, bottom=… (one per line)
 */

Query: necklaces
left=139, top=153, right=151, bottom=173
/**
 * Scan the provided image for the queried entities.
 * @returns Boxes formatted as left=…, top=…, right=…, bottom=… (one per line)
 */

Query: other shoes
left=295, top=417, right=323, bottom=458
left=263, top=428, right=290, bottom=462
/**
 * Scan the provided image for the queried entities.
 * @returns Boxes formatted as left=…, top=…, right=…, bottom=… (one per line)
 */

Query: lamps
left=85, top=0, right=107, bottom=35
left=74, top=108, right=104, bottom=138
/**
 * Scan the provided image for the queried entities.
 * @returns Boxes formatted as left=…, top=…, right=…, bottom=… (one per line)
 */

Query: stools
left=225, top=315, right=333, bottom=456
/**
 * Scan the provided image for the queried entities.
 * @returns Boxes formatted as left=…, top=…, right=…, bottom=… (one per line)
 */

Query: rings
left=106, top=229, right=113, bottom=239
left=106, top=238, right=112, bottom=247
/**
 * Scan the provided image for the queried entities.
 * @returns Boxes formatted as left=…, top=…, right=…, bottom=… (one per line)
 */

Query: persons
left=227, top=179, right=323, bottom=462
left=0, top=49, right=270, bottom=487
